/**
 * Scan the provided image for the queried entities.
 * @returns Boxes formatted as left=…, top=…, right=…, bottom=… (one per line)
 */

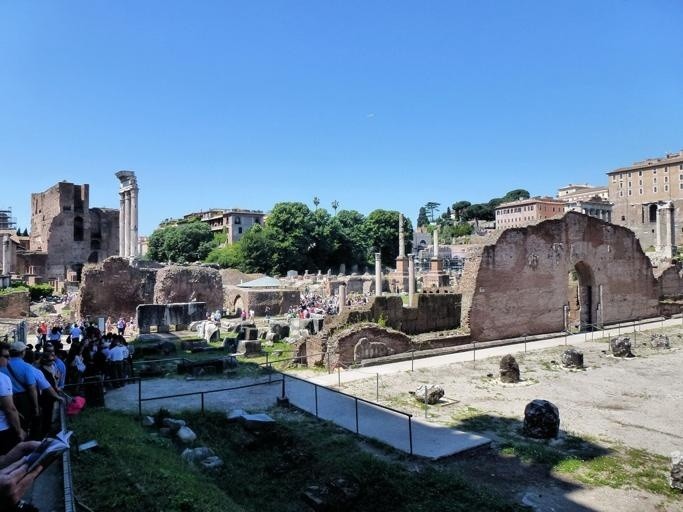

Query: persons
left=1, top=317, right=135, bottom=512
left=206, top=292, right=366, bottom=324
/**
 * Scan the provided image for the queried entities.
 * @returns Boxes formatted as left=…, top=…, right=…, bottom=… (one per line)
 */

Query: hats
left=10, top=341, right=30, bottom=351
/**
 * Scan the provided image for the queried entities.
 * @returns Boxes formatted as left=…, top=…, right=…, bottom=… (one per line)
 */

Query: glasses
left=0, top=355, right=9, bottom=359
